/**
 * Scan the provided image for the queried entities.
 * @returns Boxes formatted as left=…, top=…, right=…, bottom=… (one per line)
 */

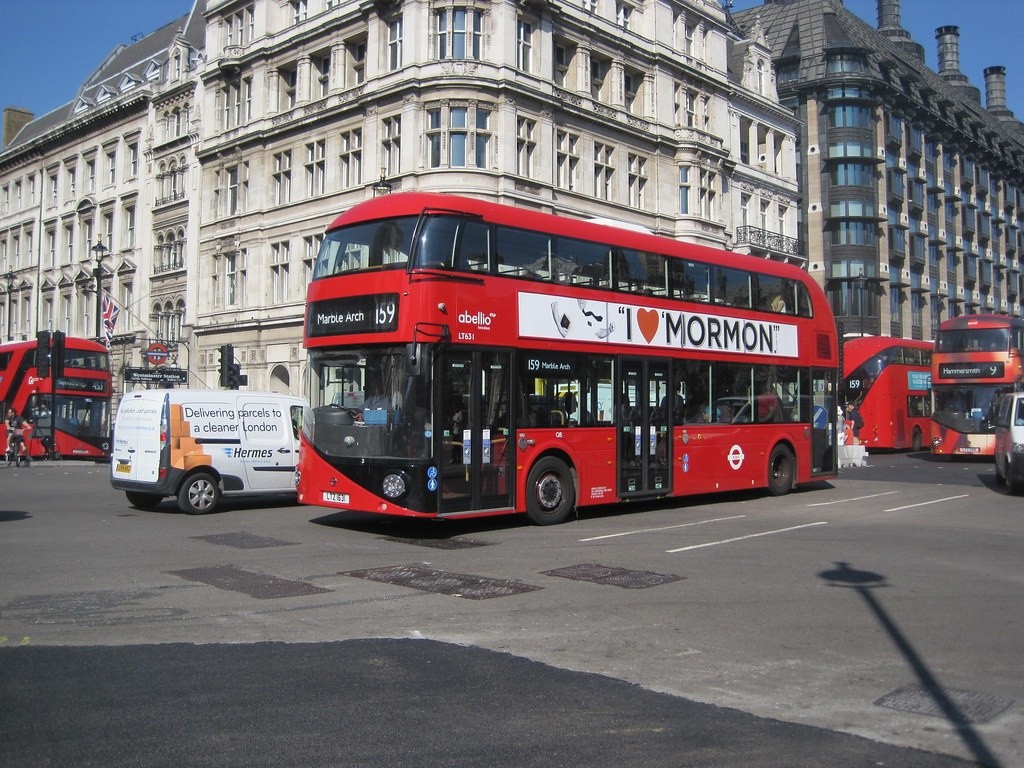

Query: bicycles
left=5, top=425, right=31, bottom=468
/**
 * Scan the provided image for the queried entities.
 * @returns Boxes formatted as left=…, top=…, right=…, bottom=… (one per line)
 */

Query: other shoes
left=26, top=456, right=33, bottom=461
left=5, top=447, right=13, bottom=454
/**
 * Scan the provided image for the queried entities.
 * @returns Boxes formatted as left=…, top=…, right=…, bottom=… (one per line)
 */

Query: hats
left=845, top=420, right=852, bottom=425
left=846, top=401, right=853, bottom=405
left=837, top=406, right=843, bottom=414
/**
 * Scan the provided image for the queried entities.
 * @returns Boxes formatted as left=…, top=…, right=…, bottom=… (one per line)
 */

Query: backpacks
left=852, top=411, right=864, bottom=429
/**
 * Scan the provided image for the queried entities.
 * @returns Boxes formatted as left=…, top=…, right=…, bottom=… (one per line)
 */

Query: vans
left=991, top=393, right=1024, bottom=492
left=106, top=389, right=329, bottom=516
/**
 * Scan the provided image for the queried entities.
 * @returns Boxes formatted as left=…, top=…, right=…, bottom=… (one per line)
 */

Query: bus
left=0, top=334, right=112, bottom=458
left=296, top=190, right=840, bottom=529
left=924, top=311, right=1024, bottom=459
left=844, top=335, right=942, bottom=453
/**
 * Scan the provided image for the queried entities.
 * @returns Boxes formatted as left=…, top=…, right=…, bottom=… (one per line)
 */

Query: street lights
left=3, top=263, right=19, bottom=342
left=90, top=232, right=109, bottom=344
left=852, top=272, right=868, bottom=338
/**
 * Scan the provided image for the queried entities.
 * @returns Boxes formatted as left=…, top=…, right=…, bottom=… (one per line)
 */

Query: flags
left=100, top=290, right=121, bottom=351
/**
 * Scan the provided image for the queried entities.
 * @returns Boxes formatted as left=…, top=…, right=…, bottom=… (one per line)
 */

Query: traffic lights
left=217, top=345, right=234, bottom=388
left=228, top=363, right=240, bottom=390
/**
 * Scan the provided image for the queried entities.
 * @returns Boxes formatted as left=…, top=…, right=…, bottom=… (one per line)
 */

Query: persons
left=837, top=402, right=865, bottom=445
left=660, top=378, right=779, bottom=424
left=5, top=408, right=33, bottom=462
left=354, top=379, right=391, bottom=421
